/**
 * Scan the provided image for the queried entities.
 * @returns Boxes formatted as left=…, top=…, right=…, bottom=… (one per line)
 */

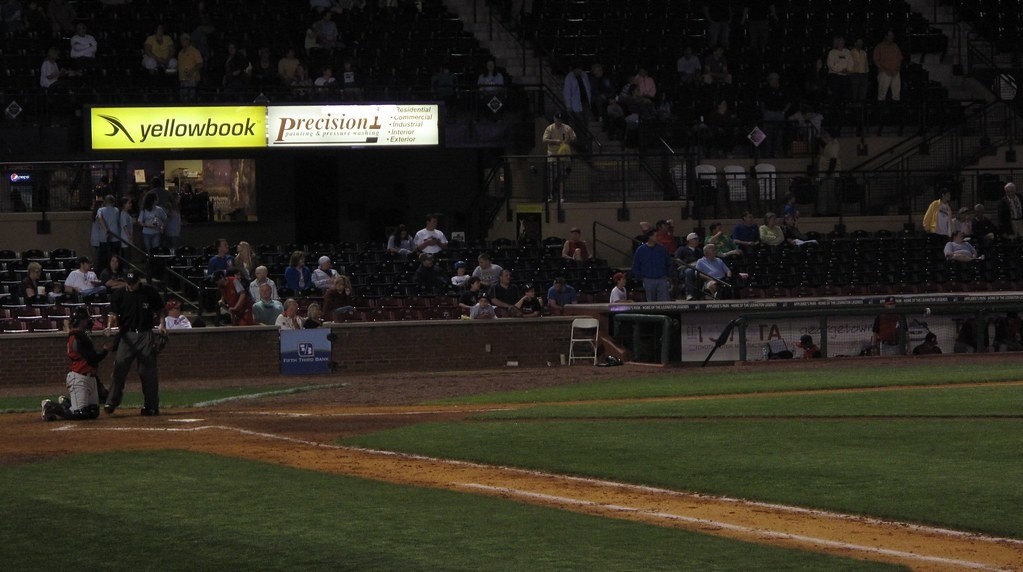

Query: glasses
left=961, top=210, right=968, bottom=214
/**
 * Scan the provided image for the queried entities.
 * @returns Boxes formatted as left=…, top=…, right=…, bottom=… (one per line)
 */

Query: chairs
left=568, top=318, right=600, bottom=368
left=670, top=230, right=1023, bottom=300
left=0, top=0, right=1023, bottom=164
left=0, top=236, right=647, bottom=333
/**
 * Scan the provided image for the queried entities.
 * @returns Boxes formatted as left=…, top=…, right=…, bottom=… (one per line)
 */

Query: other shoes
left=686, top=294, right=693, bottom=301
left=58, top=396, right=71, bottom=411
left=41, top=398, right=54, bottom=422
left=141, top=406, right=160, bottom=416
left=104, top=404, right=114, bottom=413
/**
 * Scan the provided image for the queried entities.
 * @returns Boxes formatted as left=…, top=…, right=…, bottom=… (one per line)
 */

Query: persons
left=0, top=0, right=422, bottom=102
left=158, top=300, right=192, bottom=329
left=476, top=0, right=901, bottom=201
left=207, top=239, right=284, bottom=324
left=798, top=296, right=1023, bottom=361
left=548, top=277, right=578, bottom=309
left=284, top=251, right=354, bottom=315
left=99, top=255, right=127, bottom=289
left=632, top=194, right=801, bottom=301
left=19, top=263, right=41, bottom=298
left=103, top=268, right=167, bottom=416
left=276, top=298, right=324, bottom=340
left=90, top=168, right=194, bottom=265
left=388, top=213, right=544, bottom=319
left=921, top=182, right=1023, bottom=262
left=64, top=257, right=106, bottom=297
left=562, top=228, right=593, bottom=260
left=610, top=273, right=634, bottom=303
left=47, top=282, right=62, bottom=298
left=41, top=308, right=114, bottom=422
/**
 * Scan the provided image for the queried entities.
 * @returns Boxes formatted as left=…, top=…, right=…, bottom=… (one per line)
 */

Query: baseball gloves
left=156, top=333, right=169, bottom=352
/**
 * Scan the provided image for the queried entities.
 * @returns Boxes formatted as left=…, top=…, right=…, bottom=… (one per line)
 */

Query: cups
left=78, top=296, right=83, bottom=303
left=2, top=263, right=7, bottom=270
left=4, top=285, right=10, bottom=293
left=19, top=297, right=24, bottom=305
left=35, top=308, right=40, bottom=316
left=95, top=307, right=100, bottom=314
left=43, top=252, right=49, bottom=258
left=46, top=272, right=50, bottom=280
left=170, top=248, right=175, bottom=256
left=16, top=253, right=20, bottom=259
left=60, top=262, right=63, bottom=269
left=204, top=269, right=208, bottom=276
left=65, top=308, right=70, bottom=316
left=51, top=321, right=57, bottom=329
left=5, top=309, right=10, bottom=318
left=21, top=321, right=26, bottom=330
left=37, top=286, right=45, bottom=295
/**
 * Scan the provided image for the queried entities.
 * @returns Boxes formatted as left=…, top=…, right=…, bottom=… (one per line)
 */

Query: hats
left=78, top=256, right=89, bottom=263
left=613, top=272, right=627, bottom=282
left=801, top=334, right=813, bottom=344
left=455, top=261, right=466, bottom=269
left=686, top=233, right=698, bottom=240
left=127, top=269, right=138, bottom=284
left=884, top=296, right=895, bottom=302
left=522, top=283, right=535, bottom=292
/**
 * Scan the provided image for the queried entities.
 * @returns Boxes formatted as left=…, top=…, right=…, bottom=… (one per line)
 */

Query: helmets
left=70, top=305, right=87, bottom=325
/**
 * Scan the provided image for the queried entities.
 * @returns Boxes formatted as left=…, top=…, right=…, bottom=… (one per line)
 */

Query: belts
left=1010, top=217, right=1023, bottom=221
